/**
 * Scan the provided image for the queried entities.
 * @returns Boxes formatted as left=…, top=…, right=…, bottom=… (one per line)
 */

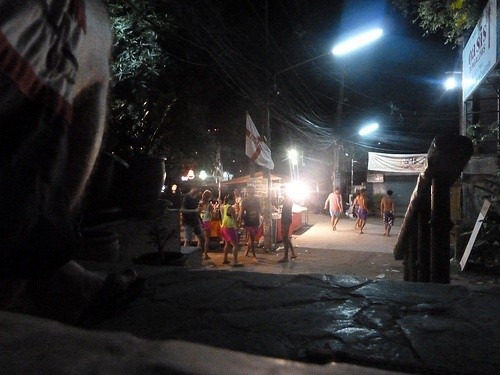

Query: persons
left=381, top=189, right=395, bottom=237
left=324, top=186, right=343, bottom=231
left=352, top=189, right=370, bottom=234
left=179, top=180, right=261, bottom=267
left=277, top=185, right=298, bottom=263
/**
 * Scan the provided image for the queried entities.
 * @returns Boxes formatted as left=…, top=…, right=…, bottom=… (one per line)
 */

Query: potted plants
left=137, top=194, right=188, bottom=266
left=59, top=193, right=120, bottom=264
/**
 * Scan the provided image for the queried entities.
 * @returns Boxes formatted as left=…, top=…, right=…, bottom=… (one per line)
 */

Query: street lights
left=332, top=121, right=379, bottom=193
left=262, top=27, right=385, bottom=252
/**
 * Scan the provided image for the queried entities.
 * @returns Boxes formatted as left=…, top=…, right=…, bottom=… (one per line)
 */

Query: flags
left=245, top=110, right=274, bottom=169
